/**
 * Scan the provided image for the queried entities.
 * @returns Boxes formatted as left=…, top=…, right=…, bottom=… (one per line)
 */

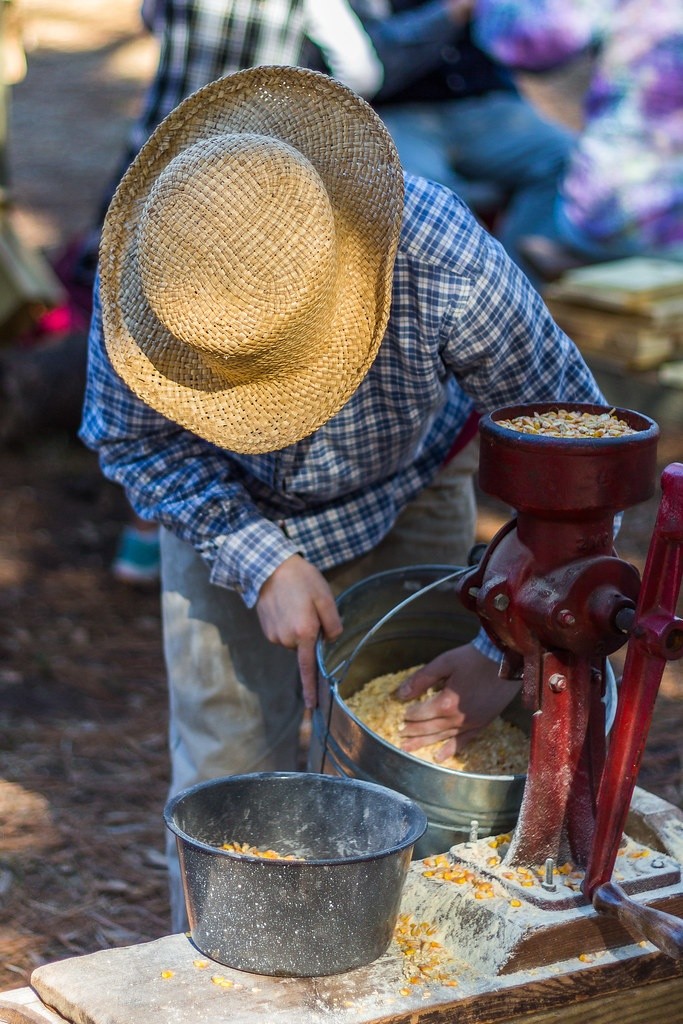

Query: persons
left=470, top=0, right=682, bottom=264
left=78, top=0, right=385, bottom=286
left=298, top=0, right=583, bottom=295
left=80, top=64, right=614, bottom=936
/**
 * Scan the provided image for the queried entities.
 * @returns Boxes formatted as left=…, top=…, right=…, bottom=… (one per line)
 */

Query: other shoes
left=113, top=522, right=159, bottom=579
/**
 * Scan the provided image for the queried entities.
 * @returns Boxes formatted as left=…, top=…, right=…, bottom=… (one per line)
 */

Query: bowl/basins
left=163, top=771, right=427, bottom=977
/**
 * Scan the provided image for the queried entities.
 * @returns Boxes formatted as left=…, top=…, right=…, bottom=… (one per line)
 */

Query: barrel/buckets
left=304, top=561, right=617, bottom=858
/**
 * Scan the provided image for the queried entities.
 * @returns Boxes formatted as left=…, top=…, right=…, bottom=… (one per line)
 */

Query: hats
left=98, top=67, right=405, bottom=453
